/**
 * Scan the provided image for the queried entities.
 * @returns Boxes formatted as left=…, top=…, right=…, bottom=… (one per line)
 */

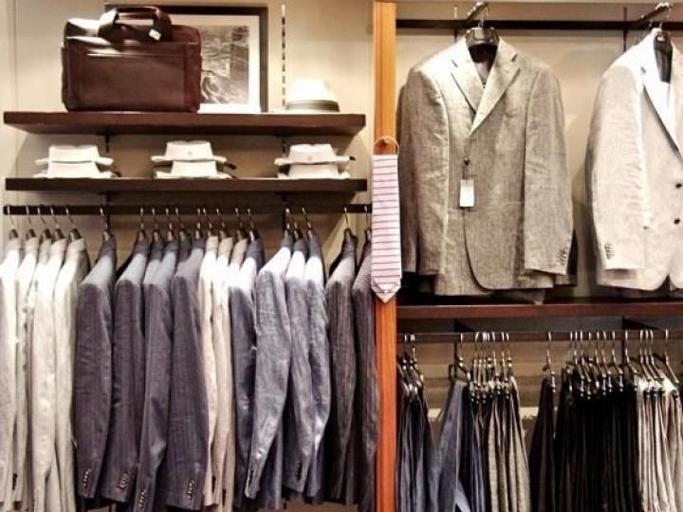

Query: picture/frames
left=104, top=4, right=268, bottom=114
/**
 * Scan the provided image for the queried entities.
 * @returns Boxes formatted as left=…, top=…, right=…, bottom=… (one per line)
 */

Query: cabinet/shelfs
left=0, top=2, right=683, bottom=512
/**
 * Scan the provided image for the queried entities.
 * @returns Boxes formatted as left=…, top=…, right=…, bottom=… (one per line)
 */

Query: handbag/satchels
left=61, top=5, right=201, bottom=112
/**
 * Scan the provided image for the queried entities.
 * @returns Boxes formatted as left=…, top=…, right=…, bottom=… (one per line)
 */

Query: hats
left=275, top=80, right=338, bottom=114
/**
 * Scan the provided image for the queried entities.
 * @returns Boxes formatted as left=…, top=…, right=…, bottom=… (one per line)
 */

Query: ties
left=371, top=136, right=402, bottom=304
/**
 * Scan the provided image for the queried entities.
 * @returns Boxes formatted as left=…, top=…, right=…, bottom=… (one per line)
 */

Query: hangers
left=397, top=328, right=681, bottom=402
left=635, top=2, right=672, bottom=61
left=464, top=1, right=502, bottom=49
